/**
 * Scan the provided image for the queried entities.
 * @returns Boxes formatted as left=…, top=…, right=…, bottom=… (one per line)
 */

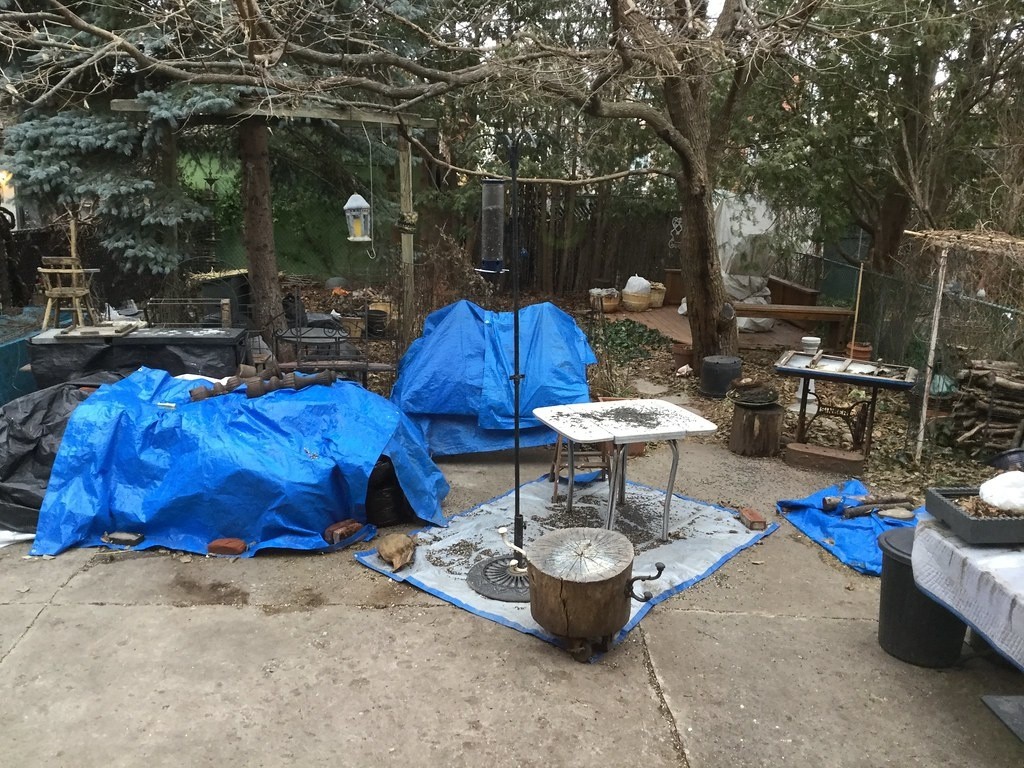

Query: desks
left=531, top=397, right=722, bottom=545
left=775, top=349, right=919, bottom=464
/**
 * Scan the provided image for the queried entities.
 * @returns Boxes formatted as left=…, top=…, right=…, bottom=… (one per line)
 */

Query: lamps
left=343, top=191, right=374, bottom=242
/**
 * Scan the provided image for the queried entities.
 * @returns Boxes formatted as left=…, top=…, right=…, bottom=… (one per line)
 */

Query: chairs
left=38, top=255, right=102, bottom=330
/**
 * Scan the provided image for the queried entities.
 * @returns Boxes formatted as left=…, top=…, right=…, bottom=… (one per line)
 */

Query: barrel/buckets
left=877, top=527, right=968, bottom=671
left=340, top=298, right=390, bottom=342
left=698, top=354, right=744, bottom=399
